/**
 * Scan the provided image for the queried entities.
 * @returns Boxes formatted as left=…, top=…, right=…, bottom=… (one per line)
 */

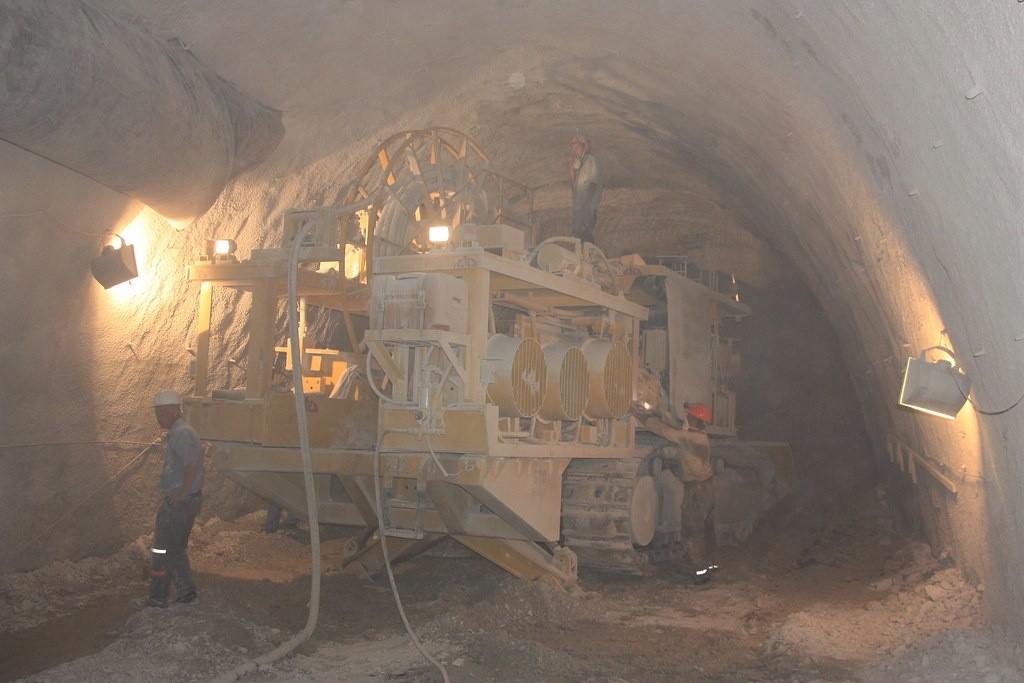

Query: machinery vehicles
left=183, top=127, right=799, bottom=598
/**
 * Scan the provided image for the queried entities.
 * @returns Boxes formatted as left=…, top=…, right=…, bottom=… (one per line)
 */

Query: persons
left=262, top=369, right=300, bottom=533
left=130, top=390, right=204, bottom=610
left=627, top=399, right=724, bottom=587
left=567, top=134, right=602, bottom=255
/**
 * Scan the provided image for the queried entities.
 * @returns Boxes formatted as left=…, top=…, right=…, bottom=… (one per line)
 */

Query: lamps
left=897, top=349, right=970, bottom=418
left=90, top=230, right=140, bottom=289
left=215, top=239, right=239, bottom=259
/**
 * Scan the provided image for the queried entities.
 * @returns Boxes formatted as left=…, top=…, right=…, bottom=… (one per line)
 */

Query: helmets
left=150, top=391, right=182, bottom=408
left=571, top=135, right=587, bottom=143
left=687, top=402, right=712, bottom=424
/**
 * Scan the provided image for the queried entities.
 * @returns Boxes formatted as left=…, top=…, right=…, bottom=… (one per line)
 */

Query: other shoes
left=702, top=559, right=721, bottom=572
left=174, top=596, right=199, bottom=608
left=693, top=566, right=710, bottom=585
left=127, top=595, right=170, bottom=611
